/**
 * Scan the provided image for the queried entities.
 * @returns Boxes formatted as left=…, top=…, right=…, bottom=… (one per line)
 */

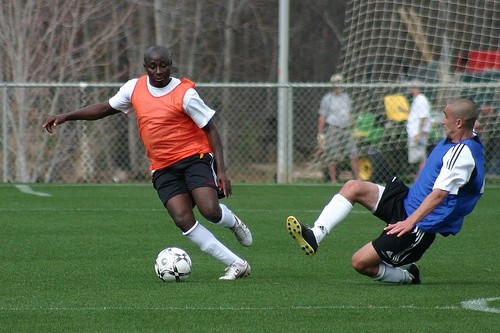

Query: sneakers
left=406, top=263, right=421, bottom=283
left=220, top=260, right=250, bottom=280
left=229, top=214, right=253, bottom=246
left=286, top=215, right=318, bottom=256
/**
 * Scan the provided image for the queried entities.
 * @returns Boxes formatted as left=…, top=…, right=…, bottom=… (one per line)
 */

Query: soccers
left=155, top=246, right=193, bottom=282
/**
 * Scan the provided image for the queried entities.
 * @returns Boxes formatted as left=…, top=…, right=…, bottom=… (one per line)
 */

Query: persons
left=313, top=72, right=361, bottom=185
left=378, top=84, right=410, bottom=184
left=285, top=91, right=486, bottom=286
left=39, top=44, right=254, bottom=281
left=407, top=78, right=433, bottom=184
